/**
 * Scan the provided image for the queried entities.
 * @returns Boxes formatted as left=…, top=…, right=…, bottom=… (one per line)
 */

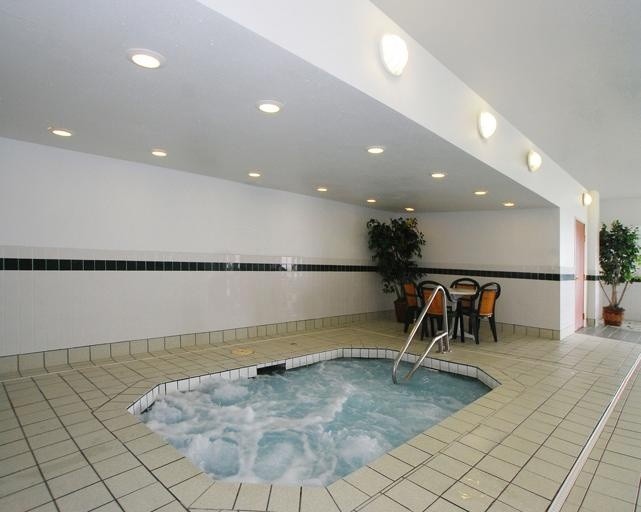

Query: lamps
left=477, top=110, right=498, bottom=139
left=527, top=150, right=542, bottom=172
left=379, top=34, right=409, bottom=77
left=582, top=192, right=593, bottom=206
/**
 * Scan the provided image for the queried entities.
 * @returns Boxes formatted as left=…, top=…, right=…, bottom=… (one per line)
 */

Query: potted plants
left=597, top=219, right=641, bottom=326
left=367, top=217, right=428, bottom=323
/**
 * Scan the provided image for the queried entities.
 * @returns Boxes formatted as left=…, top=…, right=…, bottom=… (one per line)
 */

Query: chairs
left=453, top=282, right=502, bottom=345
left=450, top=277, right=481, bottom=337
left=401, top=277, right=438, bottom=337
left=418, top=280, right=464, bottom=345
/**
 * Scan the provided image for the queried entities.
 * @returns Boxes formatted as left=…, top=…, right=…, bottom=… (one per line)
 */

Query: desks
left=434, top=288, right=481, bottom=341
